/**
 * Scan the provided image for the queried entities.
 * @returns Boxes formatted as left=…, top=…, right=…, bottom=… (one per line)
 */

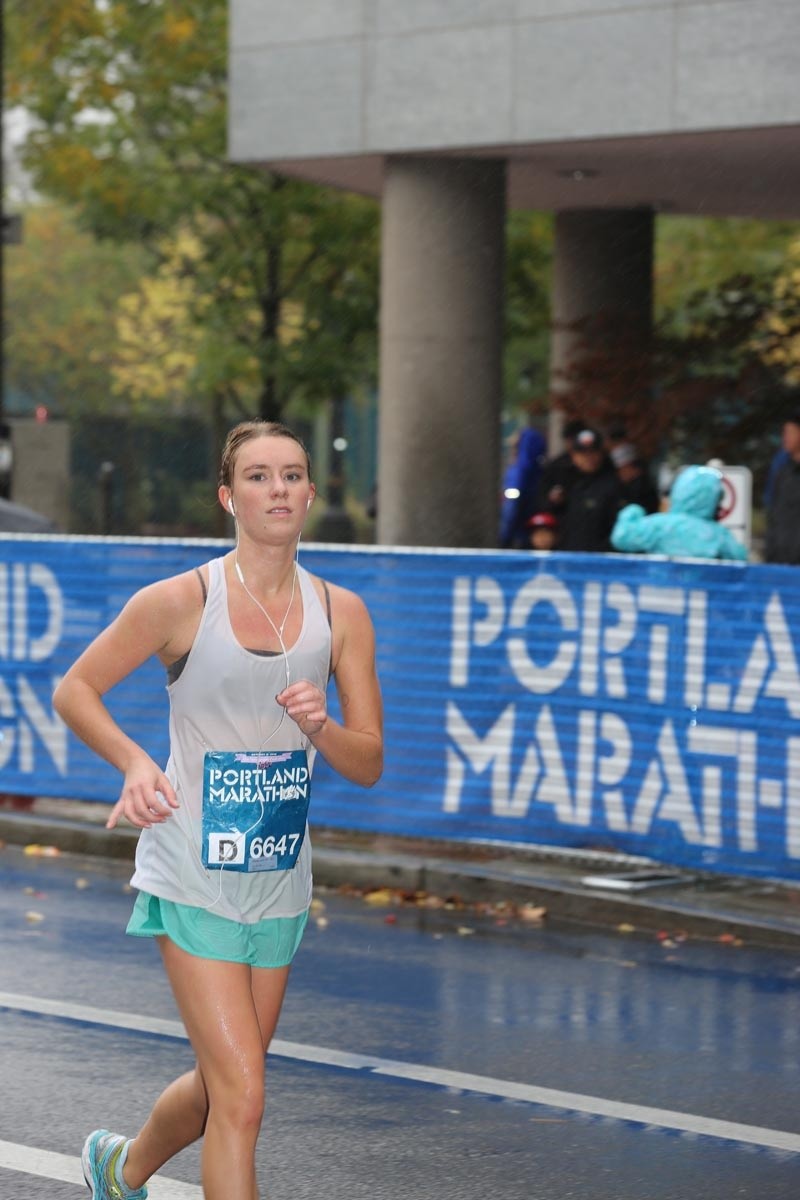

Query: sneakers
left=80, top=1129, right=149, bottom=1200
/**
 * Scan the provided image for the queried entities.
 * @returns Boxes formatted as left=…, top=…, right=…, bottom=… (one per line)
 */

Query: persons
left=49, top=422, right=384, bottom=1200
left=500, top=417, right=800, bottom=565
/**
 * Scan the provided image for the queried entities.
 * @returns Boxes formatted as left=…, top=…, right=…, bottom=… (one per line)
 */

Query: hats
left=574, top=428, right=604, bottom=453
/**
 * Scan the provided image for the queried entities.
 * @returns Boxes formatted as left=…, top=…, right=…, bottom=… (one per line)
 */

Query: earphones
left=228, top=495, right=236, bottom=515
left=306, top=499, right=310, bottom=510
